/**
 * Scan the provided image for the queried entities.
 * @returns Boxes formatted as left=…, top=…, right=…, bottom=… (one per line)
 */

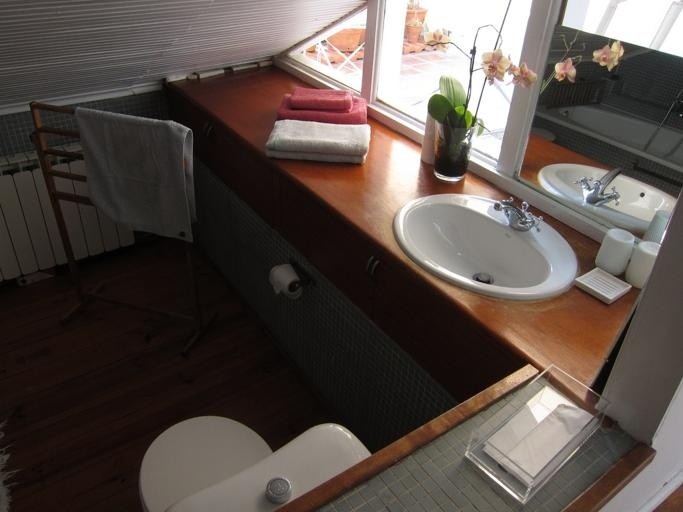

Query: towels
left=265, top=87, right=372, bottom=164
left=75, top=106, right=196, bottom=245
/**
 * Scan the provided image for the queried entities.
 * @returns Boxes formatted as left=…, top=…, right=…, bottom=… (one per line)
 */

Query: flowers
left=428, top=25, right=625, bottom=161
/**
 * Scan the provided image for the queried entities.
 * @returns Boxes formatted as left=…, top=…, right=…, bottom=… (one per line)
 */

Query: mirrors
left=502, top=0, right=683, bottom=260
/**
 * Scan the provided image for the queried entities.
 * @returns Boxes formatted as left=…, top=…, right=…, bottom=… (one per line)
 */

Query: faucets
left=492, top=196, right=544, bottom=233
left=572, top=165, right=626, bottom=207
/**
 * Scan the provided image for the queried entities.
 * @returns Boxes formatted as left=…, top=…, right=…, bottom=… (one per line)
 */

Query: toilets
left=137, top=414, right=373, bottom=512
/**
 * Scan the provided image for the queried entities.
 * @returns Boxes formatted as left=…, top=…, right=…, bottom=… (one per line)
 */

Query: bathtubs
left=536, top=101, right=683, bottom=173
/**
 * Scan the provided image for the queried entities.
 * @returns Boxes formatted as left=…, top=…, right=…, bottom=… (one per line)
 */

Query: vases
left=434, top=121, right=474, bottom=185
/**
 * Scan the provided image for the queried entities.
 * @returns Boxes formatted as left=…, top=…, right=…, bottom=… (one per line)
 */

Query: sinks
left=537, top=162, right=678, bottom=236
left=393, top=193, right=580, bottom=302
